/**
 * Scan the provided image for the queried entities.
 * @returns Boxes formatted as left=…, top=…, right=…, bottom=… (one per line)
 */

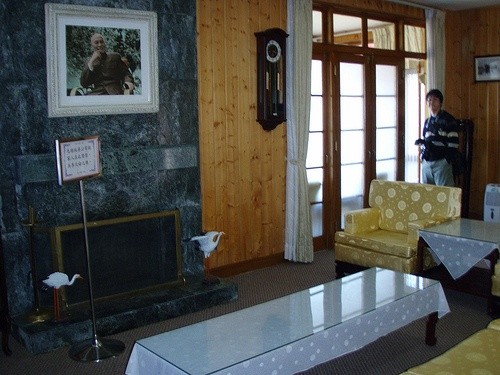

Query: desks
left=124, top=266, right=451, bottom=375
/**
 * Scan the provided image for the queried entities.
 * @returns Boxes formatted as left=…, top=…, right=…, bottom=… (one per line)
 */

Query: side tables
left=417, top=218, right=500, bottom=316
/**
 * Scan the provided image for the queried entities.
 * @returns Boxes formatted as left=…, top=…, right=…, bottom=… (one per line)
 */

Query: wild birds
left=43, top=272, right=84, bottom=321
left=191, top=230, right=225, bottom=281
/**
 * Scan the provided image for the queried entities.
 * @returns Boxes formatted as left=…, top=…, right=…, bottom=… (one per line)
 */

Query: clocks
left=253, top=27, right=290, bottom=131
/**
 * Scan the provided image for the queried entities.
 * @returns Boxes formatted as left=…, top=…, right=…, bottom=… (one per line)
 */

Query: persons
left=420, top=89, right=459, bottom=187
left=80, top=33, right=135, bottom=95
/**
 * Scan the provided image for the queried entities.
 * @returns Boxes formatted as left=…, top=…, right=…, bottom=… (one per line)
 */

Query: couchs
left=334, top=179, right=462, bottom=280
left=399, top=319, right=500, bottom=375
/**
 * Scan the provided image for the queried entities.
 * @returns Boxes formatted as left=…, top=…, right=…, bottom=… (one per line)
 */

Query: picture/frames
left=44, top=3, right=160, bottom=118
left=473, top=54, right=500, bottom=83
